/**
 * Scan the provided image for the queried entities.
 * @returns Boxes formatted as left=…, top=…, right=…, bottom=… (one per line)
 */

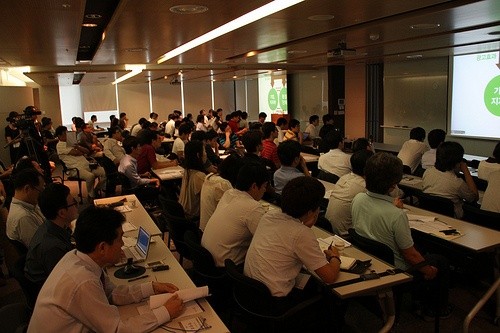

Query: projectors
left=327, top=49, right=357, bottom=58
left=172, top=81, right=181, bottom=85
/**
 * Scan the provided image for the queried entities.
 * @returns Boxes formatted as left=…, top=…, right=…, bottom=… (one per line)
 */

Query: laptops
left=114, top=226, right=151, bottom=267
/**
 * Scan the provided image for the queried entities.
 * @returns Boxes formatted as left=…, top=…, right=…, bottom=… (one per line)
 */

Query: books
left=338, top=255, right=355, bottom=270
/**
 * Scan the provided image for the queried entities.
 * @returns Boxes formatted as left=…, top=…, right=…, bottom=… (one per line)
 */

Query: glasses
left=61, top=198, right=78, bottom=209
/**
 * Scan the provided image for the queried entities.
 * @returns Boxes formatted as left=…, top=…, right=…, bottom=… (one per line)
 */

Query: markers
left=395, top=126, right=400, bottom=127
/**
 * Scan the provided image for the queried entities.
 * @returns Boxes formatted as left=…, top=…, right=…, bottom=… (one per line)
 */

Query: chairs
left=0, top=141, right=500, bottom=333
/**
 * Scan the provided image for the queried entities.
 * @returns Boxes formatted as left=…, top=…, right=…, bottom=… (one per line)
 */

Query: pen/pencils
left=328, top=240, right=334, bottom=250
left=128, top=275, right=149, bottom=282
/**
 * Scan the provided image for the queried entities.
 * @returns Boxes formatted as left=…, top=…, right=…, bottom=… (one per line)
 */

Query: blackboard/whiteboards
left=380, top=72, right=448, bottom=134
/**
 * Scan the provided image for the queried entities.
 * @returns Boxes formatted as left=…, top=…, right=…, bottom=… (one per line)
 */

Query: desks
left=151, top=155, right=185, bottom=201
left=93, top=194, right=163, bottom=237
left=299, top=152, right=318, bottom=163
left=371, top=140, right=401, bottom=155
left=460, top=153, right=489, bottom=177
left=302, top=226, right=414, bottom=333
left=398, top=173, right=484, bottom=221
left=105, top=235, right=230, bottom=333
left=399, top=204, right=500, bottom=333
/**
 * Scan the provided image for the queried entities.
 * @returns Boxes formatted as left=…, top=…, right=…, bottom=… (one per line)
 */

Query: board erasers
left=402, top=126, right=408, bottom=128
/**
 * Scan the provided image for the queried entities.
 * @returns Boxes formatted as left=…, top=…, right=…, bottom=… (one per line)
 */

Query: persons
left=24, top=183, right=80, bottom=292
left=200, top=163, right=270, bottom=270
left=271, top=139, right=310, bottom=197
left=353, top=138, right=419, bottom=207
left=5, top=170, right=46, bottom=249
left=26, top=205, right=184, bottom=333
left=318, top=130, right=353, bottom=178
left=477, top=144, right=500, bottom=212
left=326, top=149, right=373, bottom=241
left=421, top=129, right=447, bottom=169
left=398, top=127, right=428, bottom=177
left=420, top=142, right=478, bottom=219
left=350, top=152, right=445, bottom=320
left=0, top=105, right=340, bottom=238
left=244, top=175, right=347, bottom=333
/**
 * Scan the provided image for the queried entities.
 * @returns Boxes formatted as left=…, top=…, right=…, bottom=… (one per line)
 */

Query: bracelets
left=330, top=256, right=342, bottom=264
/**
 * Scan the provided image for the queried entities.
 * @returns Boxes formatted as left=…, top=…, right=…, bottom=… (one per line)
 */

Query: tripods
left=3, top=130, right=40, bottom=165
